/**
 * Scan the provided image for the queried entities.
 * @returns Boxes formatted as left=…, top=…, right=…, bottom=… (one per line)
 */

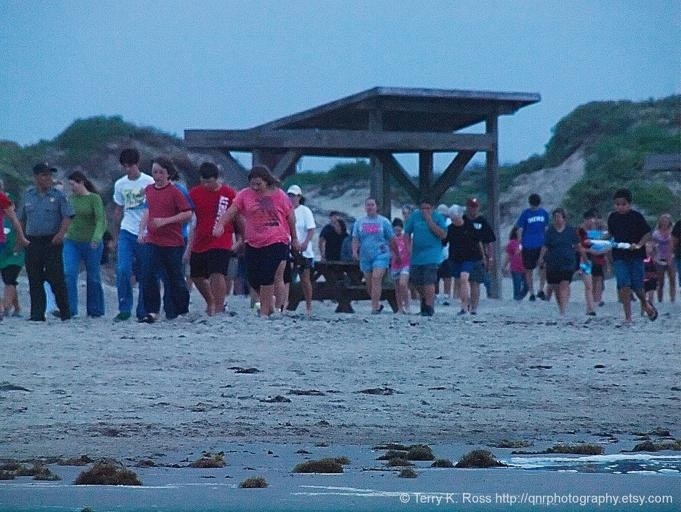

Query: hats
left=467, top=198, right=480, bottom=208
left=287, top=184, right=303, bottom=196
left=33, top=164, right=57, bottom=173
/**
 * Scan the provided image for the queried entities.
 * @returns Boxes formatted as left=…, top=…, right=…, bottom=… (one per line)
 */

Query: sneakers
left=529, top=291, right=546, bottom=302
left=421, top=303, right=435, bottom=317
left=112, top=310, right=132, bottom=323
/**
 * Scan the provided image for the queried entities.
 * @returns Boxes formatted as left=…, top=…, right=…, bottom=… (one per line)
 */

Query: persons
left=0, top=149, right=681, bottom=324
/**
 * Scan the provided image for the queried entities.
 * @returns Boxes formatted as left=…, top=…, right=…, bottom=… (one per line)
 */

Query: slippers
left=645, top=299, right=659, bottom=321
left=138, top=313, right=156, bottom=325
